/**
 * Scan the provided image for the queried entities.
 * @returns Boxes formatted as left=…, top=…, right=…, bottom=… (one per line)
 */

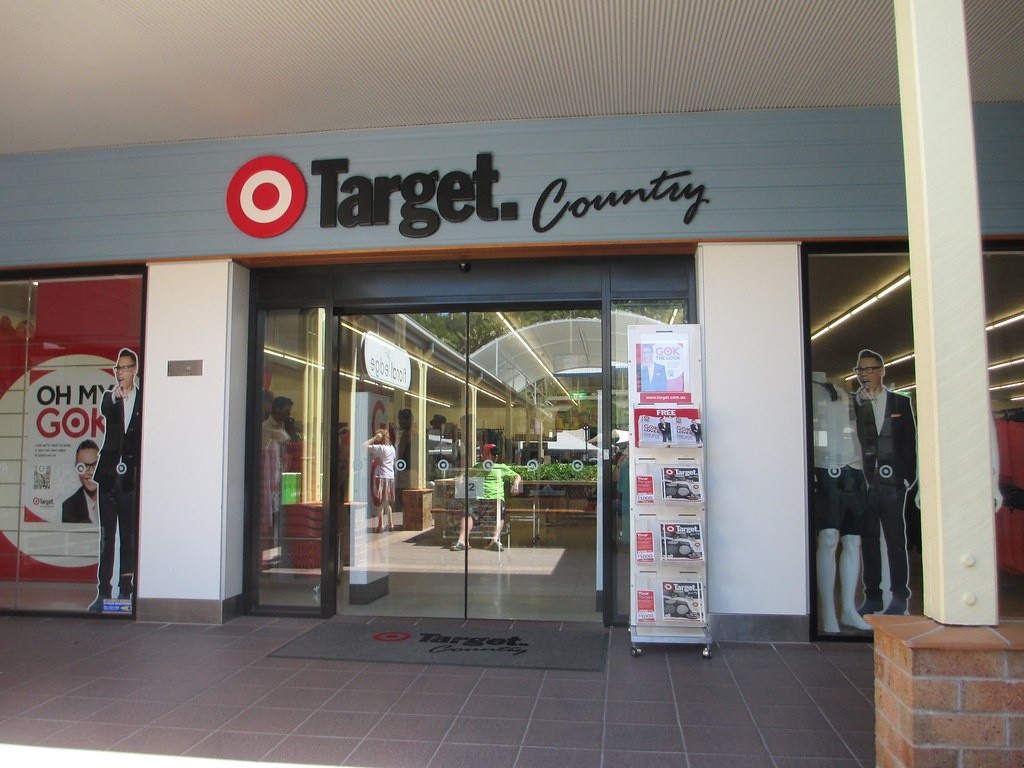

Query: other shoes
left=89, top=592, right=112, bottom=614
left=371, top=527, right=385, bottom=534
left=857, top=599, right=883, bottom=617
left=385, top=526, right=395, bottom=533
left=883, top=597, right=907, bottom=616
left=117, top=593, right=130, bottom=600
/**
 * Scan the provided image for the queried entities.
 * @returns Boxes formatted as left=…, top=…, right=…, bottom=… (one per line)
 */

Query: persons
left=857, top=352, right=917, bottom=618
left=59, top=439, right=99, bottom=523
left=658, top=418, right=671, bottom=442
left=690, top=419, right=703, bottom=444
left=812, top=370, right=873, bottom=634
left=450, top=444, right=521, bottom=552
left=261, top=397, right=294, bottom=450
left=637, top=345, right=667, bottom=391
left=362, top=428, right=396, bottom=533
left=914, top=417, right=1003, bottom=512
left=88, top=350, right=140, bottom=610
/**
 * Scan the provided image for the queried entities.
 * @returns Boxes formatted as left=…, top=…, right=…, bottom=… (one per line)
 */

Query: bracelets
left=373, top=437, right=377, bottom=440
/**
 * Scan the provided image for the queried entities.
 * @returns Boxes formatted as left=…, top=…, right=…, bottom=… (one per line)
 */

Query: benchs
left=431, top=505, right=597, bottom=549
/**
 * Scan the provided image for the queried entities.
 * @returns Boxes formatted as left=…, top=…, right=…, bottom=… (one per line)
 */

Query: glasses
left=85, top=461, right=98, bottom=472
left=113, top=364, right=136, bottom=373
left=853, top=365, right=883, bottom=375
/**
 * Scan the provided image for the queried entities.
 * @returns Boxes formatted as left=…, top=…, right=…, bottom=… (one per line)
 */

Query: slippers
left=449, top=541, right=472, bottom=551
left=483, top=541, right=505, bottom=552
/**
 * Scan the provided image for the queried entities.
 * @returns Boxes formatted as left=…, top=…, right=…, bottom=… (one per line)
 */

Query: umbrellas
left=547, top=431, right=600, bottom=453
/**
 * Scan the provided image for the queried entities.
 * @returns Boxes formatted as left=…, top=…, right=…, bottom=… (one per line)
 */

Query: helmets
left=481, top=443, right=496, bottom=462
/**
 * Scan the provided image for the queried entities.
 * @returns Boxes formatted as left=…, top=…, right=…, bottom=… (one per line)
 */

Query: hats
left=430, top=415, right=446, bottom=424
